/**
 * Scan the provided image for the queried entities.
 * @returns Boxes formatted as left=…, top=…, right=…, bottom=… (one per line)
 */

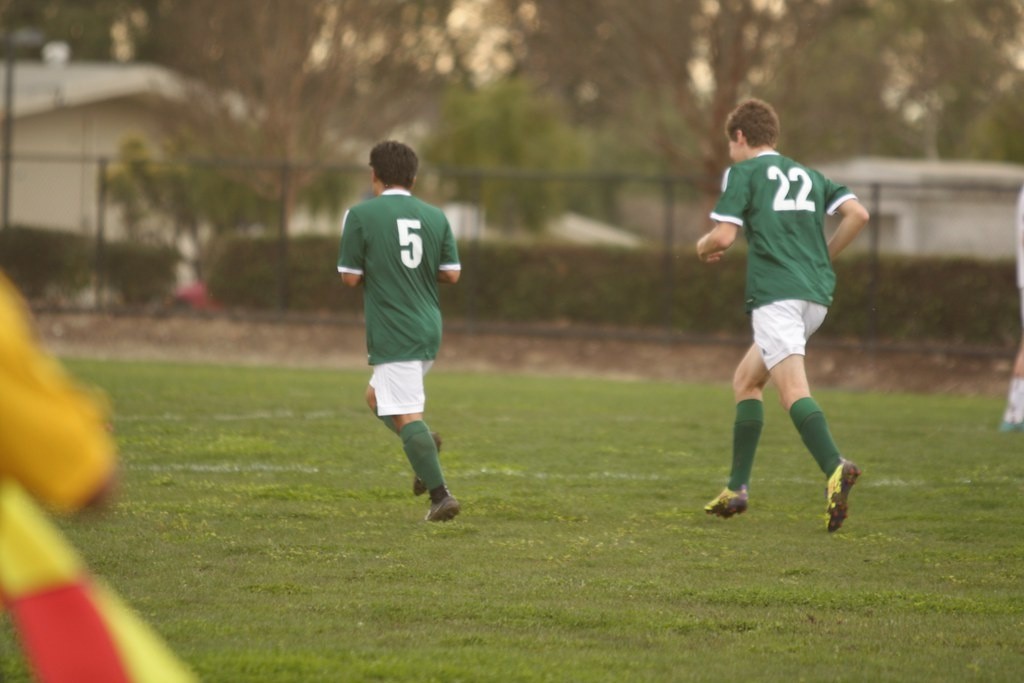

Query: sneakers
left=702, top=483, right=750, bottom=516
left=412, top=431, right=442, bottom=494
left=824, top=457, right=862, bottom=532
left=422, top=495, right=460, bottom=522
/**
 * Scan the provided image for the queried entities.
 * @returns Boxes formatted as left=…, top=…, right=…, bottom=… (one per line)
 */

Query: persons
left=332, top=138, right=463, bottom=520
left=692, top=99, right=874, bottom=531
